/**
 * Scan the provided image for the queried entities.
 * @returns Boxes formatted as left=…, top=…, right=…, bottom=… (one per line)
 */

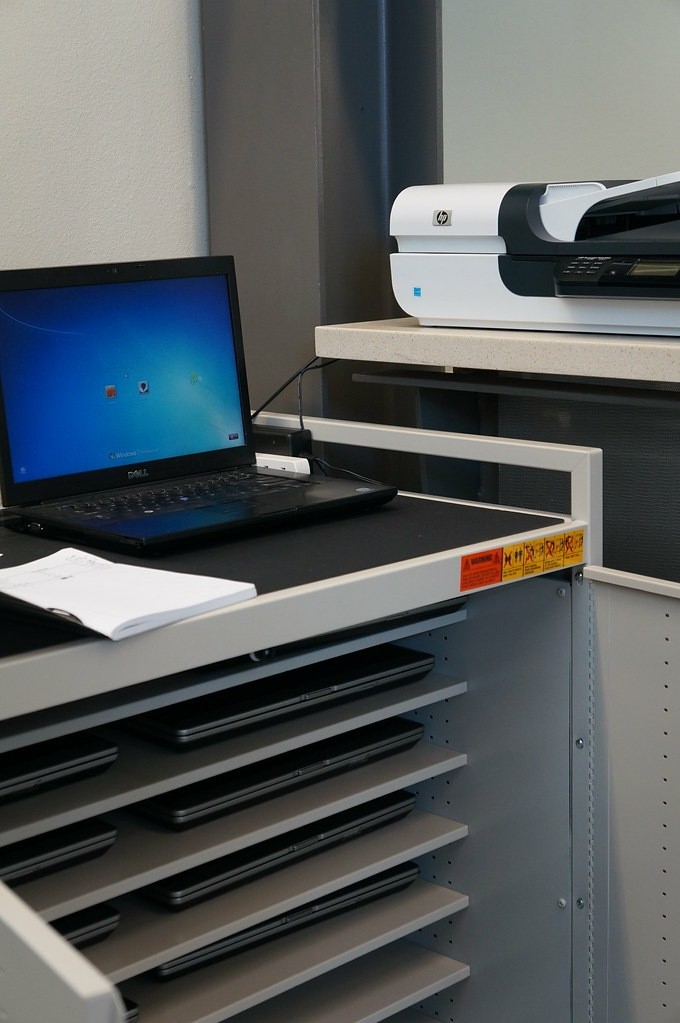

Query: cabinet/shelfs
left=0, top=409, right=680, bottom=1022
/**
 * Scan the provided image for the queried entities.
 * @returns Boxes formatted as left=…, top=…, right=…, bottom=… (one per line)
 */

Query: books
left=1, top=544, right=260, bottom=642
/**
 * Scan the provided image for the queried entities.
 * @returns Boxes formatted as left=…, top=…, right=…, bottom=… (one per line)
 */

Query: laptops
left=0, top=254, right=398, bottom=560
left=1, top=642, right=438, bottom=1022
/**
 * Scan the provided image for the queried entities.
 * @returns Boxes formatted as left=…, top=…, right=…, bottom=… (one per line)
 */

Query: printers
left=388, top=167, right=680, bottom=338
